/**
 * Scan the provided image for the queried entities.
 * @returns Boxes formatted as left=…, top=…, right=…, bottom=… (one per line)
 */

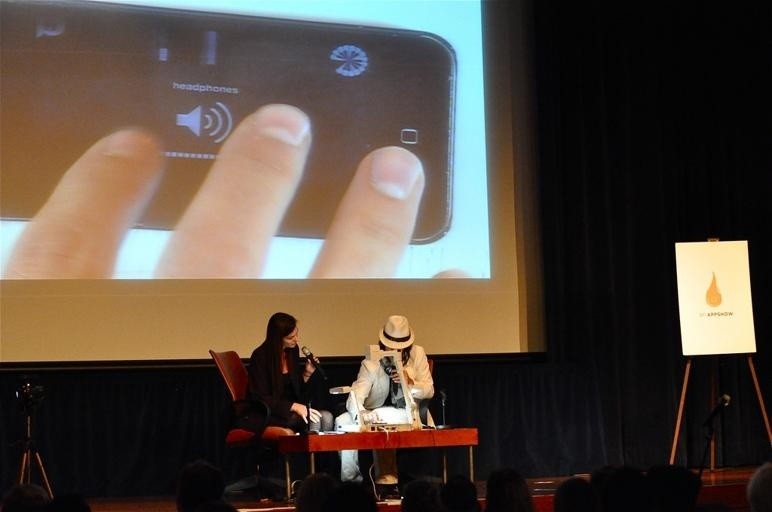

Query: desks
left=278, top=428, right=479, bottom=504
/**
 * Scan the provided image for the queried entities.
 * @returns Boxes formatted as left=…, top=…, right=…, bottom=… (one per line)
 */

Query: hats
left=379, top=315, right=414, bottom=349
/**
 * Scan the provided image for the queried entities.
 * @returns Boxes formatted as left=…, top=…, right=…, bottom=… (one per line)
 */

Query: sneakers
left=379, top=485, right=400, bottom=499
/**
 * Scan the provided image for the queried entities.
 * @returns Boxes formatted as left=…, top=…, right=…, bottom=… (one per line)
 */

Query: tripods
left=16, top=414, right=55, bottom=501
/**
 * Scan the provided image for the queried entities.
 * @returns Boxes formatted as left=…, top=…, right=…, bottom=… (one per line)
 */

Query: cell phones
left=0, top=0, right=458, bottom=246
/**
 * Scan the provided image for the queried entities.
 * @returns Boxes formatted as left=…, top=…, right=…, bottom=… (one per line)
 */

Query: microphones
left=702, top=394, right=730, bottom=428
left=391, top=360, right=398, bottom=395
left=302, top=346, right=328, bottom=382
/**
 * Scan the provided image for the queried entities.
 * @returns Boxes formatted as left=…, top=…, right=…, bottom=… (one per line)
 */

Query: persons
left=1, top=102, right=474, bottom=282
left=0, top=484, right=51, bottom=512
left=332, top=316, right=438, bottom=487
left=175, top=461, right=238, bottom=511
left=44, top=493, right=92, bottom=511
left=242, top=311, right=336, bottom=501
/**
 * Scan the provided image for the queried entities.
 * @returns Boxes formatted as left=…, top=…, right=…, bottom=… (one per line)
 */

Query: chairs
left=428, top=358, right=447, bottom=484
left=209, top=349, right=316, bottom=505
